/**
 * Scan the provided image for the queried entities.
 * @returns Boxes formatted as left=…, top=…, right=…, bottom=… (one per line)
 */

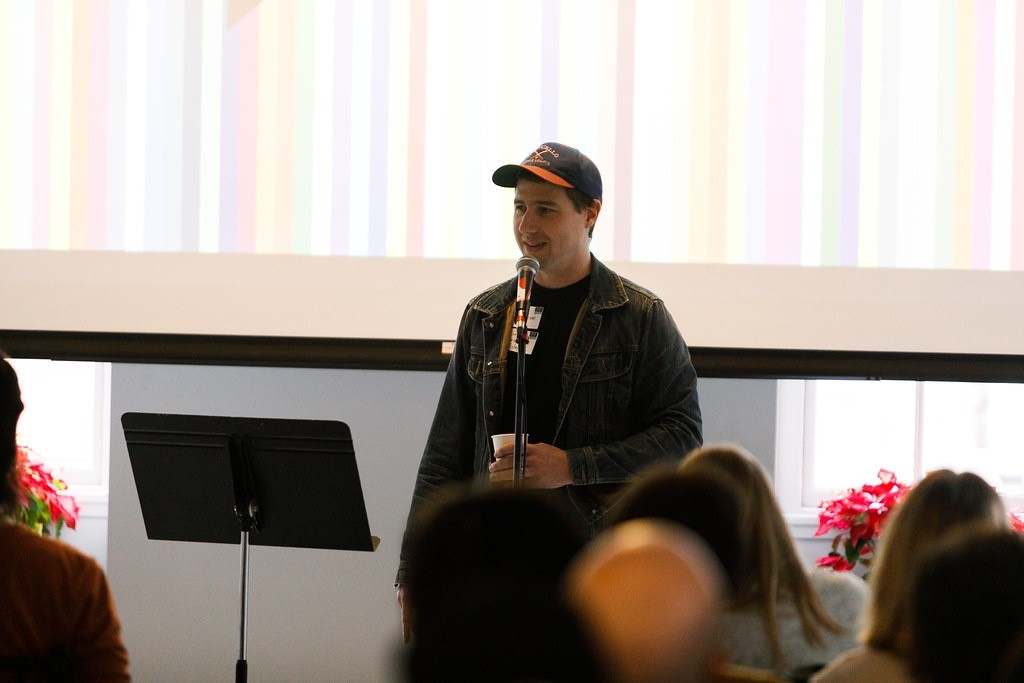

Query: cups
left=491, top=433, right=529, bottom=461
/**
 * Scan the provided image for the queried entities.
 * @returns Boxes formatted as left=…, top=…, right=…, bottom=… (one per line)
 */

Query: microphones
left=516, top=255, right=540, bottom=337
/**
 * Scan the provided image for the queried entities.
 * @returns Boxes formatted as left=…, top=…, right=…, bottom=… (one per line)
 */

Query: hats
left=492, top=142, right=602, bottom=201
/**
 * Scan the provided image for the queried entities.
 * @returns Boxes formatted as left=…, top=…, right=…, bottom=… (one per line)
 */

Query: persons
left=0, top=354, right=132, bottom=683
left=394, top=441, right=1024, bottom=683
left=394, top=142, right=704, bottom=645
